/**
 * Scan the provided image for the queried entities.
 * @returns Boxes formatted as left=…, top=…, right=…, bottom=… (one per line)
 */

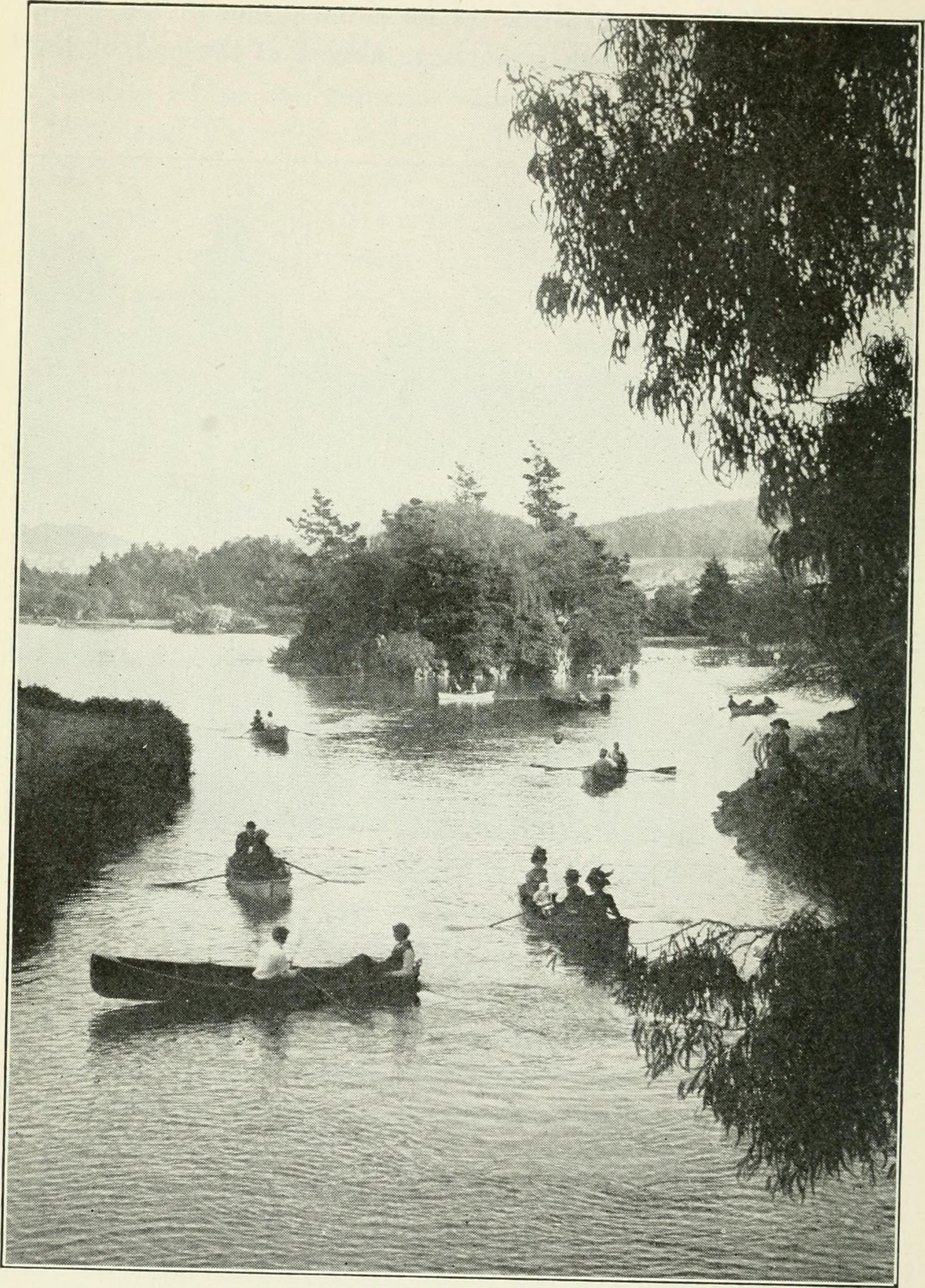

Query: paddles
left=611, top=764, right=677, bottom=775
left=151, top=867, right=231, bottom=887
left=277, top=859, right=368, bottom=885
left=604, top=910, right=693, bottom=925
left=445, top=901, right=554, bottom=931
left=295, top=968, right=371, bottom=1028
left=238, top=726, right=254, bottom=739
left=718, top=707, right=729, bottom=711
left=275, top=725, right=316, bottom=737
left=529, top=763, right=593, bottom=773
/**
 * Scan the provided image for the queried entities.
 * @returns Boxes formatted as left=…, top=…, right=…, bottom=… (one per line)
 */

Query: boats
left=730, top=703, right=778, bottom=714
left=89, top=951, right=423, bottom=1005
left=227, top=855, right=290, bottom=901
left=519, top=888, right=629, bottom=953
left=582, top=768, right=627, bottom=787
left=437, top=691, right=494, bottom=699
left=541, top=692, right=600, bottom=709
left=253, top=725, right=288, bottom=744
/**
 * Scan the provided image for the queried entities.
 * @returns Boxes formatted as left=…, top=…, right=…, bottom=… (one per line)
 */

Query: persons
left=234, top=821, right=283, bottom=872
left=593, top=742, right=626, bottom=777
left=345, top=923, right=414, bottom=981
left=251, top=926, right=303, bottom=991
left=729, top=696, right=776, bottom=708
left=252, top=709, right=277, bottom=731
left=526, top=845, right=624, bottom=922
